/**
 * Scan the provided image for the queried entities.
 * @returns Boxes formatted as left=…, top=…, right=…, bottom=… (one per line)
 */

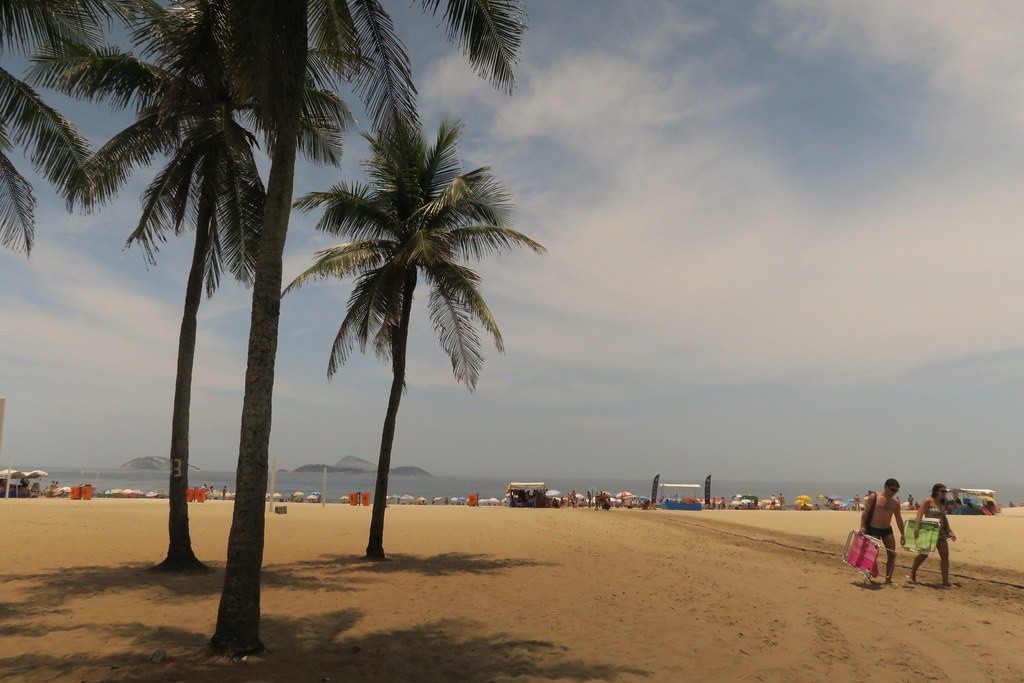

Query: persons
left=199, top=483, right=214, bottom=501
left=222, top=486, right=226, bottom=500
left=770, top=493, right=776, bottom=510
left=908, top=483, right=962, bottom=590
left=567, top=489, right=609, bottom=511
left=49, top=480, right=59, bottom=498
left=510, top=489, right=545, bottom=508
left=778, top=493, right=785, bottom=510
left=858, top=478, right=906, bottom=586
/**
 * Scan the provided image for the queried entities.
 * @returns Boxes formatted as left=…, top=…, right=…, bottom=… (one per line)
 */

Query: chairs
left=900, top=515, right=941, bottom=552
left=841, top=530, right=898, bottom=583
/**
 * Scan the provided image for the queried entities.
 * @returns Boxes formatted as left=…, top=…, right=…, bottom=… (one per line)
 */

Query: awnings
left=659, top=483, right=701, bottom=500
left=945, top=487, right=997, bottom=504
left=505, top=482, right=546, bottom=509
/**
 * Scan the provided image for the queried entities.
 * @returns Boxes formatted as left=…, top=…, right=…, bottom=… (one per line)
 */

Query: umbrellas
left=793, top=495, right=813, bottom=510
left=615, top=490, right=650, bottom=508
left=401, top=494, right=414, bottom=505
left=816, top=494, right=859, bottom=512
left=544, top=489, right=560, bottom=508
left=730, top=492, right=782, bottom=510
left=307, top=495, right=317, bottom=503
left=292, top=491, right=304, bottom=502
left=272, top=492, right=282, bottom=502
left=312, top=491, right=321, bottom=503
left=0, top=468, right=48, bottom=496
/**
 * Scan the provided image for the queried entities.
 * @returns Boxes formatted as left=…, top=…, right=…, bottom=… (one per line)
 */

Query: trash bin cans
left=71, top=486, right=81, bottom=500
left=349, top=492, right=358, bottom=506
left=187, top=489, right=194, bottom=503
left=195, top=489, right=206, bottom=503
left=362, top=492, right=371, bottom=506
left=468, top=493, right=478, bottom=506
left=82, top=485, right=92, bottom=500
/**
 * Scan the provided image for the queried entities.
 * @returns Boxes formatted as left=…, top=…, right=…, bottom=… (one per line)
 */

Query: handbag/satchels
left=943, top=517, right=952, bottom=538
left=864, top=492, right=877, bottom=534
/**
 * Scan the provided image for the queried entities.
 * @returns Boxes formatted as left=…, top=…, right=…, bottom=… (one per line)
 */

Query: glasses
left=889, top=487, right=897, bottom=492
left=939, top=490, right=947, bottom=493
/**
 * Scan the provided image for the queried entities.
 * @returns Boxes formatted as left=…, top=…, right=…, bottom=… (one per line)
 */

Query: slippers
left=944, top=585, right=958, bottom=589
left=906, top=575, right=916, bottom=583
